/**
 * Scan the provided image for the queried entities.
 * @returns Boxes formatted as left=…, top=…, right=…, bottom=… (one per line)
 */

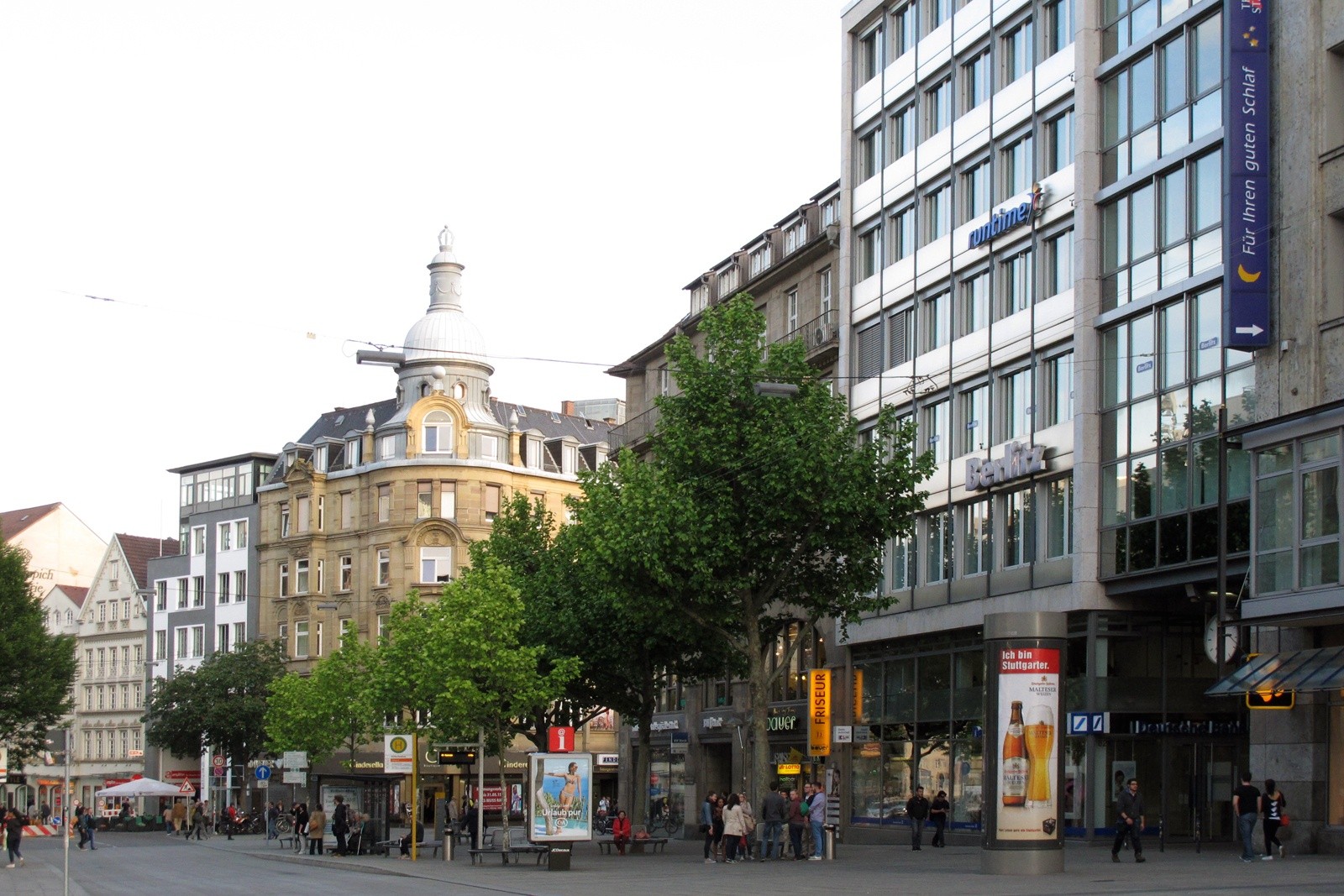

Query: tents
left=94, top=777, right=195, bottom=831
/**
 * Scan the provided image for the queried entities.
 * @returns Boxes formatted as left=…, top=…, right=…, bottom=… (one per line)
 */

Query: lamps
left=1203, top=612, right=1242, bottom=667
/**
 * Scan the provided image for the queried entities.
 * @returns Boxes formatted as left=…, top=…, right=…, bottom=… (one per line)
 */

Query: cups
left=1024, top=705, right=1054, bottom=807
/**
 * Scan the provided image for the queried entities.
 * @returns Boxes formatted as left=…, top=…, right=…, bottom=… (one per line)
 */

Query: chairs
left=757, top=823, right=785, bottom=857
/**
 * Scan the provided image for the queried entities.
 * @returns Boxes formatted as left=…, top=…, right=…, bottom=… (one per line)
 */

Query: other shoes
left=932, top=841, right=945, bottom=847
left=705, top=852, right=823, bottom=864
left=618, top=849, right=625, bottom=855
left=399, top=854, right=409, bottom=859
left=18, top=857, right=23, bottom=863
left=1136, top=852, right=1145, bottom=862
left=1261, top=855, right=1273, bottom=860
left=7, top=864, right=15, bottom=868
left=912, top=846, right=921, bottom=852
left=1112, top=855, right=1120, bottom=863
left=1239, top=856, right=1253, bottom=863
left=1279, top=846, right=1285, bottom=858
left=294, top=850, right=357, bottom=857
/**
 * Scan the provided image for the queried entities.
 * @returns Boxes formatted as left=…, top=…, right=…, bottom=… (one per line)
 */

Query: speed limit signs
left=213, top=755, right=225, bottom=767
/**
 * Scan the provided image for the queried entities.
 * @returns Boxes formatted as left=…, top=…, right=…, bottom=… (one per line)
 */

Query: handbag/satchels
left=740, top=835, right=746, bottom=847
left=1280, top=814, right=1290, bottom=827
left=635, top=829, right=646, bottom=838
left=193, top=812, right=202, bottom=823
left=310, top=820, right=317, bottom=831
left=332, top=824, right=337, bottom=836
left=304, top=822, right=310, bottom=833
left=751, top=818, right=757, bottom=825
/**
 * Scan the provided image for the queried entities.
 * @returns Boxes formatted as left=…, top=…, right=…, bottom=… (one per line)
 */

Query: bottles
left=1003, top=701, right=1030, bottom=806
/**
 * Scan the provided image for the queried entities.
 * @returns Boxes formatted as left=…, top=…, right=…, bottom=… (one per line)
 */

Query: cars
left=866, top=795, right=910, bottom=820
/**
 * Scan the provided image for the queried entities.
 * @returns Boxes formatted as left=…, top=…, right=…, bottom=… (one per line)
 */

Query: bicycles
left=710, top=834, right=747, bottom=855
left=646, top=813, right=679, bottom=834
left=593, top=813, right=615, bottom=836
left=214, top=806, right=295, bottom=835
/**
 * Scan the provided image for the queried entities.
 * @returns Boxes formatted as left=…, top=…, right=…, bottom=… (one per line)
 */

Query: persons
left=120, top=798, right=132, bottom=817
left=1260, top=779, right=1287, bottom=860
left=398, top=815, right=425, bottom=859
left=264, top=793, right=371, bottom=856
left=1233, top=770, right=1261, bottom=862
left=545, top=762, right=583, bottom=835
left=28, top=802, right=36, bottom=826
left=163, top=798, right=214, bottom=841
left=5, top=808, right=25, bottom=869
left=226, top=802, right=236, bottom=840
left=40, top=800, right=50, bottom=825
left=447, top=795, right=474, bottom=830
left=907, top=786, right=929, bottom=852
left=930, top=791, right=950, bottom=848
left=461, top=803, right=489, bottom=850
left=613, top=810, right=631, bottom=854
left=598, top=795, right=612, bottom=822
left=74, top=802, right=100, bottom=851
left=1111, top=779, right=1146, bottom=863
left=699, top=780, right=829, bottom=864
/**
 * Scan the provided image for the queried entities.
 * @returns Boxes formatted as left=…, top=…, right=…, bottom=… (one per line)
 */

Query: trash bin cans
left=822, top=825, right=836, bottom=860
left=443, top=828, right=455, bottom=861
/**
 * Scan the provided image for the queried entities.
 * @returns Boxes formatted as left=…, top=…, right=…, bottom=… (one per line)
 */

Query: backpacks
left=799, top=801, right=808, bottom=816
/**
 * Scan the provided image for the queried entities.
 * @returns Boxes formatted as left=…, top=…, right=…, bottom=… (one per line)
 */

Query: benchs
left=278, top=837, right=297, bottom=848
left=598, top=839, right=668, bottom=853
left=383, top=829, right=549, bottom=866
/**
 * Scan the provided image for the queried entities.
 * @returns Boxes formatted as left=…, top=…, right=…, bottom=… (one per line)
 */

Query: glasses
left=573, top=767, right=578, bottom=769
left=804, top=786, right=809, bottom=788
